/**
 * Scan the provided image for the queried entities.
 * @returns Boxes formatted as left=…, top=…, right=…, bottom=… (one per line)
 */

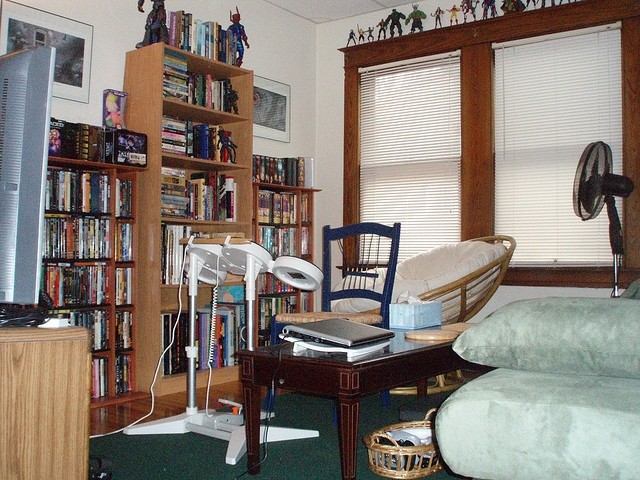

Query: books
left=90, top=354, right=134, bottom=397
left=259, top=293, right=310, bottom=330
left=161, top=115, right=217, bottom=160
left=41, top=219, right=133, bottom=262
left=159, top=224, right=210, bottom=285
left=159, top=285, right=247, bottom=375
left=160, top=165, right=238, bottom=222
left=44, top=169, right=134, bottom=218
left=48, top=310, right=134, bottom=353
left=163, top=47, right=225, bottom=112
left=259, top=334, right=274, bottom=350
left=257, top=226, right=308, bottom=258
left=253, top=154, right=314, bottom=188
left=38, top=265, right=133, bottom=306
left=64, top=123, right=106, bottom=162
left=257, top=273, right=299, bottom=294
left=254, top=190, right=310, bottom=224
left=172, top=9, right=229, bottom=63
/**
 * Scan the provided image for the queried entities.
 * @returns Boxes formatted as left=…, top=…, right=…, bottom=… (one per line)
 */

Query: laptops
left=282, top=318, right=395, bottom=347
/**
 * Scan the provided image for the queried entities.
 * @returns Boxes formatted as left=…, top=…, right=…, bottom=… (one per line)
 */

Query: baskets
left=363, top=408, right=444, bottom=480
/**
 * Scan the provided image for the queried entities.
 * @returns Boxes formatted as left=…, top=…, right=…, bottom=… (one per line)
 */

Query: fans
left=572, top=141, right=634, bottom=267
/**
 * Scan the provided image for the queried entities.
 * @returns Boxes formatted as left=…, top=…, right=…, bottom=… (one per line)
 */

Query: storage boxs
left=104, top=128, right=148, bottom=167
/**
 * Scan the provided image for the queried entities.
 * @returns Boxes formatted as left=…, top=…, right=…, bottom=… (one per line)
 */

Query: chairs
left=357, top=236, right=516, bottom=396
left=263, top=221, right=401, bottom=425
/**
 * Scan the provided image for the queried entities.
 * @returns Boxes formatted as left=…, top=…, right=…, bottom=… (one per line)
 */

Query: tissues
left=388, top=290, right=442, bottom=330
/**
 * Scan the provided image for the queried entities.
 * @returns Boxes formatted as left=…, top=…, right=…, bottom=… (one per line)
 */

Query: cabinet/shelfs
left=35, top=157, right=151, bottom=410
left=255, top=183, right=322, bottom=346
left=122, top=42, right=254, bottom=397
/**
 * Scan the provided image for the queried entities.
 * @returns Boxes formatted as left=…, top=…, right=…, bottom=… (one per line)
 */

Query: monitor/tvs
left=1, top=47, right=57, bottom=305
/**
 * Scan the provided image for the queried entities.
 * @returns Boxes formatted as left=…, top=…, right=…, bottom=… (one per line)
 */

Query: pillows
left=452, top=297, right=639, bottom=379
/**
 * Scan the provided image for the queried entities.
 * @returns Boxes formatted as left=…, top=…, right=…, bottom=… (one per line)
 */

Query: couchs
left=435, top=278, right=639, bottom=479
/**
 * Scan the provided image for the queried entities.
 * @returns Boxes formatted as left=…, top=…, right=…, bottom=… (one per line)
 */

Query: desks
left=0, top=326, right=93, bottom=479
left=234, top=322, right=476, bottom=479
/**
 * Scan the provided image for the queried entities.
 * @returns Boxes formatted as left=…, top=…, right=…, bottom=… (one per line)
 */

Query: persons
left=446, top=5, right=461, bottom=28
left=48, top=129, right=62, bottom=157
left=346, top=29, right=357, bottom=48
left=8, top=31, right=31, bottom=53
left=104, top=92, right=123, bottom=128
left=357, top=23, right=367, bottom=45
left=365, top=27, right=375, bottom=44
left=430, top=7, right=445, bottom=30
left=406, top=4, right=426, bottom=36
left=225, top=83, right=241, bottom=114
left=385, top=9, right=406, bottom=39
left=376, top=19, right=387, bottom=42
left=481, top=0, right=498, bottom=21
left=228, top=6, right=249, bottom=67
left=135, top=0, right=170, bottom=49
left=217, top=130, right=239, bottom=164
left=461, top=0, right=480, bottom=24
